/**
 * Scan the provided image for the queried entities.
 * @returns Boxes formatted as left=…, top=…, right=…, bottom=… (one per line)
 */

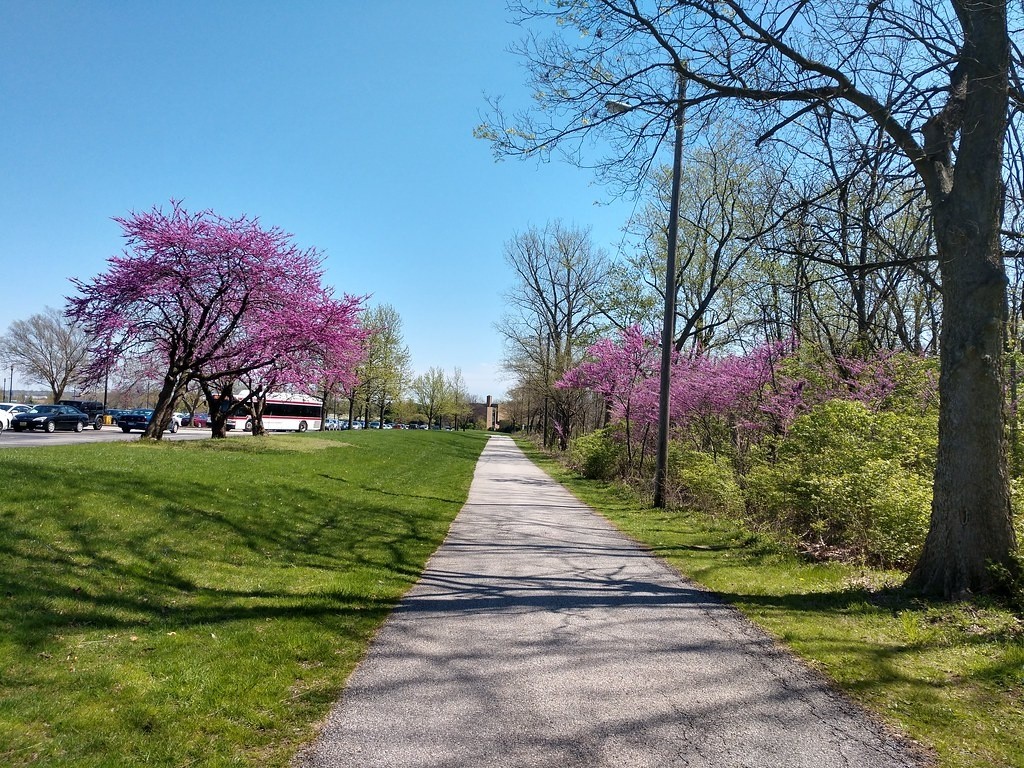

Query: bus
left=204, top=390, right=323, bottom=434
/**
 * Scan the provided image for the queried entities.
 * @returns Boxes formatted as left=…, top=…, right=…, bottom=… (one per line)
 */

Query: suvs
left=57, top=400, right=106, bottom=430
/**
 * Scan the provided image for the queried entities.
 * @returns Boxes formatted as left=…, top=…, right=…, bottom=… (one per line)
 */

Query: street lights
left=602, top=57, right=692, bottom=514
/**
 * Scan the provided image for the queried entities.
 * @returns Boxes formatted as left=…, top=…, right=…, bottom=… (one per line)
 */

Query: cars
left=325, top=418, right=455, bottom=432
left=106, top=408, right=125, bottom=424
left=11, top=403, right=89, bottom=433
left=0, top=402, right=33, bottom=431
left=171, top=412, right=186, bottom=425
left=182, top=415, right=208, bottom=428
left=117, top=410, right=178, bottom=435
